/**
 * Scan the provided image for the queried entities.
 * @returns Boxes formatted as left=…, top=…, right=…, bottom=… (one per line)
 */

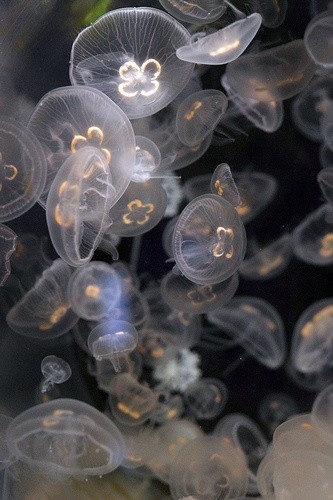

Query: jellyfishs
left=0, top=1, right=331, bottom=499
